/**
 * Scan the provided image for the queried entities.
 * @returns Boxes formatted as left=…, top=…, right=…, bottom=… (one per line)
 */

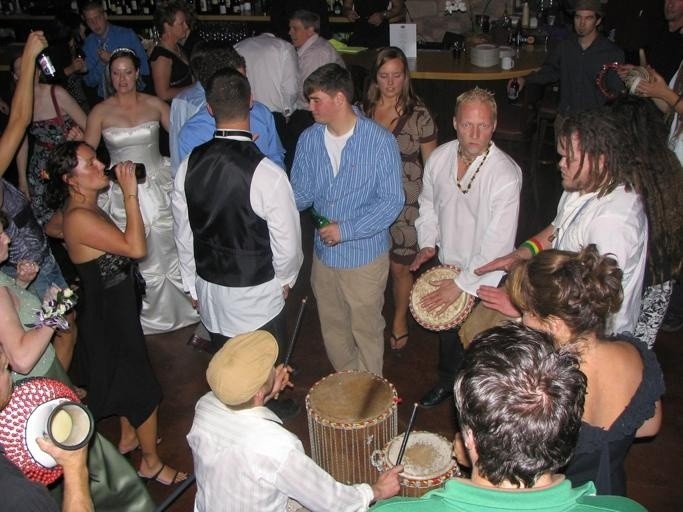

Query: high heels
left=135, top=461, right=193, bottom=488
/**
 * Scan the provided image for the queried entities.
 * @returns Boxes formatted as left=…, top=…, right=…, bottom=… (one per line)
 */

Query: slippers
left=389, top=320, right=411, bottom=352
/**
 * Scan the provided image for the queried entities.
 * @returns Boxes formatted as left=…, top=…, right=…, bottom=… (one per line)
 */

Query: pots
left=475, top=14, right=489, bottom=24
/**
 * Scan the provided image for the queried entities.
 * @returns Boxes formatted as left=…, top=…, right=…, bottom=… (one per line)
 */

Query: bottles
left=188, top=0, right=268, bottom=17
left=105, top=163, right=146, bottom=180
left=73, top=40, right=89, bottom=75
left=503, top=2, right=509, bottom=24
left=522, top=0, right=556, bottom=30
left=512, top=30, right=527, bottom=47
left=453, top=40, right=461, bottom=59
left=334, top=0, right=342, bottom=16
left=508, top=77, right=519, bottom=102
left=103, top=0, right=161, bottom=16
left=29, top=26, right=58, bottom=80
left=139, top=25, right=160, bottom=47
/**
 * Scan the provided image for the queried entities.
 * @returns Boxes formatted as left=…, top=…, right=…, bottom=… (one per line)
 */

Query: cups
left=501, top=57, right=514, bottom=70
left=43, top=401, right=94, bottom=451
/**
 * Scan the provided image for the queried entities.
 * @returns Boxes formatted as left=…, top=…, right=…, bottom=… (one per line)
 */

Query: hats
left=204, top=327, right=284, bottom=408
left=569, top=0, right=607, bottom=17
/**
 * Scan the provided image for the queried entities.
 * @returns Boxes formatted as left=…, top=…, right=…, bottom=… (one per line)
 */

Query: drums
left=408, top=264, right=475, bottom=332
left=380, top=430, right=466, bottom=498
left=305, top=370, right=398, bottom=486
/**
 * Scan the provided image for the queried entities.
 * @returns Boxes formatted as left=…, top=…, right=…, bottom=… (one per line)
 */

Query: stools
left=493, top=105, right=539, bottom=205
left=537, top=85, right=560, bottom=175
left=497, top=83, right=542, bottom=105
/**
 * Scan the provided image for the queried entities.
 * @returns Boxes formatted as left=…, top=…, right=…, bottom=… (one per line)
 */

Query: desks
left=341, top=43, right=551, bottom=145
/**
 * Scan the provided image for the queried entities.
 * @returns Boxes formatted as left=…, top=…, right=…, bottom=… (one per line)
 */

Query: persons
left=2, top=0, right=683, bottom=422
left=181, top=330, right=404, bottom=512
left=366, top=319, right=654, bottom=512
left=40, top=138, right=190, bottom=487
left=1, top=340, right=158, bottom=512
left=503, top=242, right=669, bottom=496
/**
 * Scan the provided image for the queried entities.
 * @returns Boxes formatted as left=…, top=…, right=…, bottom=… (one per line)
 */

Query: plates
left=471, top=44, right=514, bottom=67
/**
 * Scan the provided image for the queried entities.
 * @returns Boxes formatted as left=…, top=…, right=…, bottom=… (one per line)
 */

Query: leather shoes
left=417, top=383, right=453, bottom=409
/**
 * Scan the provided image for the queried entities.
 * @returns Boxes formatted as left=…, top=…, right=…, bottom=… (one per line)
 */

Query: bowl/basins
left=509, top=16, right=520, bottom=24
